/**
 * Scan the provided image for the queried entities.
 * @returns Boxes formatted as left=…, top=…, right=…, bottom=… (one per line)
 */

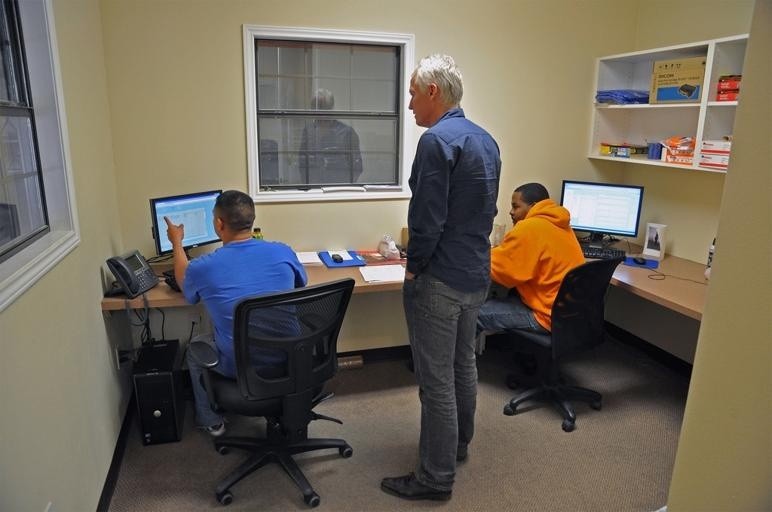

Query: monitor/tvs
left=560, top=180, right=644, bottom=248
left=149, top=190, right=223, bottom=277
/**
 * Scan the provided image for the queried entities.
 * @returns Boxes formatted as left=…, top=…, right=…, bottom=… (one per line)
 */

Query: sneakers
left=205, top=421, right=225, bottom=437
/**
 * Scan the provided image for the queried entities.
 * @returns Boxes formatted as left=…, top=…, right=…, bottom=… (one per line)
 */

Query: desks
left=101, top=250, right=411, bottom=369
left=580, top=234, right=711, bottom=379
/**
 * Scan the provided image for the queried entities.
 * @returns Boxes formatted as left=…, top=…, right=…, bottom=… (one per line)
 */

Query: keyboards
left=581, top=246, right=625, bottom=261
left=165, top=275, right=180, bottom=293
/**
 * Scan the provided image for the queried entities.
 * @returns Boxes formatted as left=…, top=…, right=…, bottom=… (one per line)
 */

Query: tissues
left=378, top=234, right=401, bottom=260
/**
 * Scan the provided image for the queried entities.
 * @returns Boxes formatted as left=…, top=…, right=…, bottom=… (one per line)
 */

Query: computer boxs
left=132, top=339, right=183, bottom=445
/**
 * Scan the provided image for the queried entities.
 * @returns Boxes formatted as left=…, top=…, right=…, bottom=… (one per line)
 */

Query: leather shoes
left=381, top=472, right=452, bottom=502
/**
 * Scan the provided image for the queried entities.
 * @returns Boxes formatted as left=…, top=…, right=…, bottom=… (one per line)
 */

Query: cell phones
left=332, top=253, right=344, bottom=263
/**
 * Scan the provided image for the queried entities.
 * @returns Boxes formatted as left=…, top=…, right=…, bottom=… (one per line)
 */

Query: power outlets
left=114, top=342, right=125, bottom=371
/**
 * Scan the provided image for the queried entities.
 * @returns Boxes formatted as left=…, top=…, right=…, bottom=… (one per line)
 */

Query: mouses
left=633, top=257, right=646, bottom=264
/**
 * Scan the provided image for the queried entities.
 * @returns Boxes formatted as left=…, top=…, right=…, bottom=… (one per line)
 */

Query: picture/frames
left=640, top=219, right=667, bottom=260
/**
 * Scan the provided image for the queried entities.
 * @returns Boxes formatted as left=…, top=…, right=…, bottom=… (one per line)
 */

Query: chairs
left=199, top=275, right=369, bottom=506
left=492, top=256, right=631, bottom=433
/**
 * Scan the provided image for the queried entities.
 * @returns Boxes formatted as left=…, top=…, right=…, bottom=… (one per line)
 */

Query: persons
left=297, top=88, right=362, bottom=182
left=476, top=182, right=589, bottom=340
left=163, top=188, right=308, bottom=437
left=379, top=52, right=499, bottom=501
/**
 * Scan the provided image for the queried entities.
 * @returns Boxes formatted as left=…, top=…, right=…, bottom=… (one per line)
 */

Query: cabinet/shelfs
left=587, top=31, right=749, bottom=174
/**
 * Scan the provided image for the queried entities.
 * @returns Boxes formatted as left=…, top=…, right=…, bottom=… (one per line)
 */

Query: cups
left=493, top=223, right=507, bottom=248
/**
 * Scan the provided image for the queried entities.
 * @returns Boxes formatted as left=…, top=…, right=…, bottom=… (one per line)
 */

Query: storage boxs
left=643, top=55, right=708, bottom=107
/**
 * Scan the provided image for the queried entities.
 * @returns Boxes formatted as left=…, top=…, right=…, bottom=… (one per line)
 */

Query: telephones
left=106, top=250, right=160, bottom=299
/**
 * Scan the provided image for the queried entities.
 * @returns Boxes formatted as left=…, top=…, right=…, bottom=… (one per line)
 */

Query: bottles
left=251, top=229, right=263, bottom=239
left=704, top=237, right=716, bottom=280
